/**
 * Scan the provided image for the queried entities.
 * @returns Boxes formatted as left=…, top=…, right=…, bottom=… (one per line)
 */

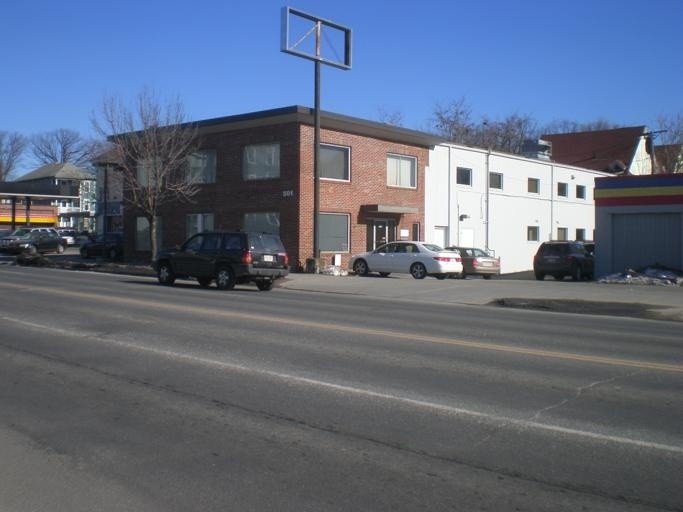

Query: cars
left=348, top=240, right=501, bottom=279
left=0, top=227, right=88, bottom=256
left=80, top=232, right=123, bottom=259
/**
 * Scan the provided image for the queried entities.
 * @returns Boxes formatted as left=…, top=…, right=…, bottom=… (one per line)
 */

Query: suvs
left=534, top=241, right=595, bottom=281
left=152, top=230, right=291, bottom=290
